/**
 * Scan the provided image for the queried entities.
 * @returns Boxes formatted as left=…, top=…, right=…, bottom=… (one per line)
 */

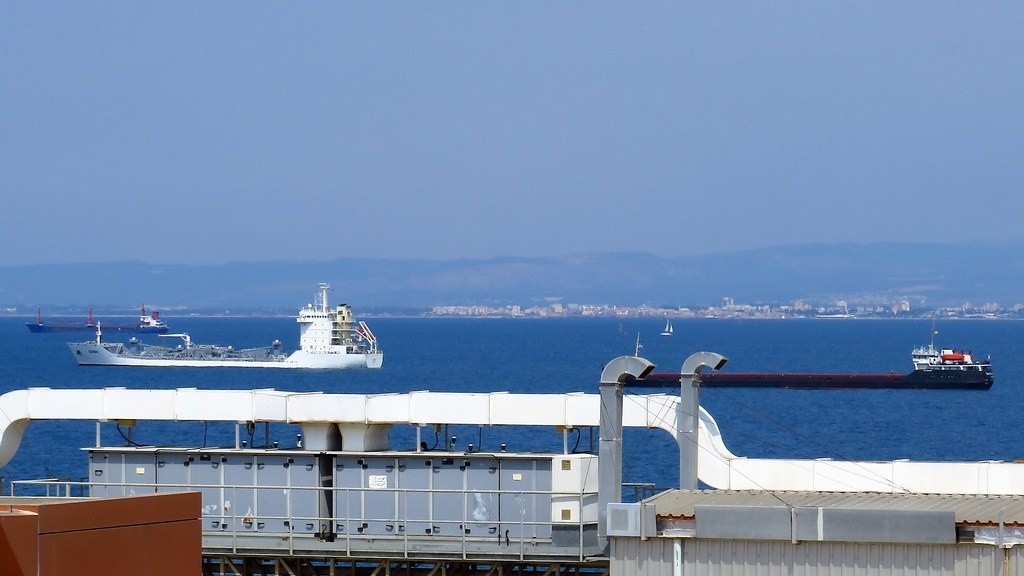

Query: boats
left=65, top=281, right=384, bottom=370
left=910, top=318, right=994, bottom=384
left=660, top=320, right=675, bottom=337
left=24, top=303, right=170, bottom=333
